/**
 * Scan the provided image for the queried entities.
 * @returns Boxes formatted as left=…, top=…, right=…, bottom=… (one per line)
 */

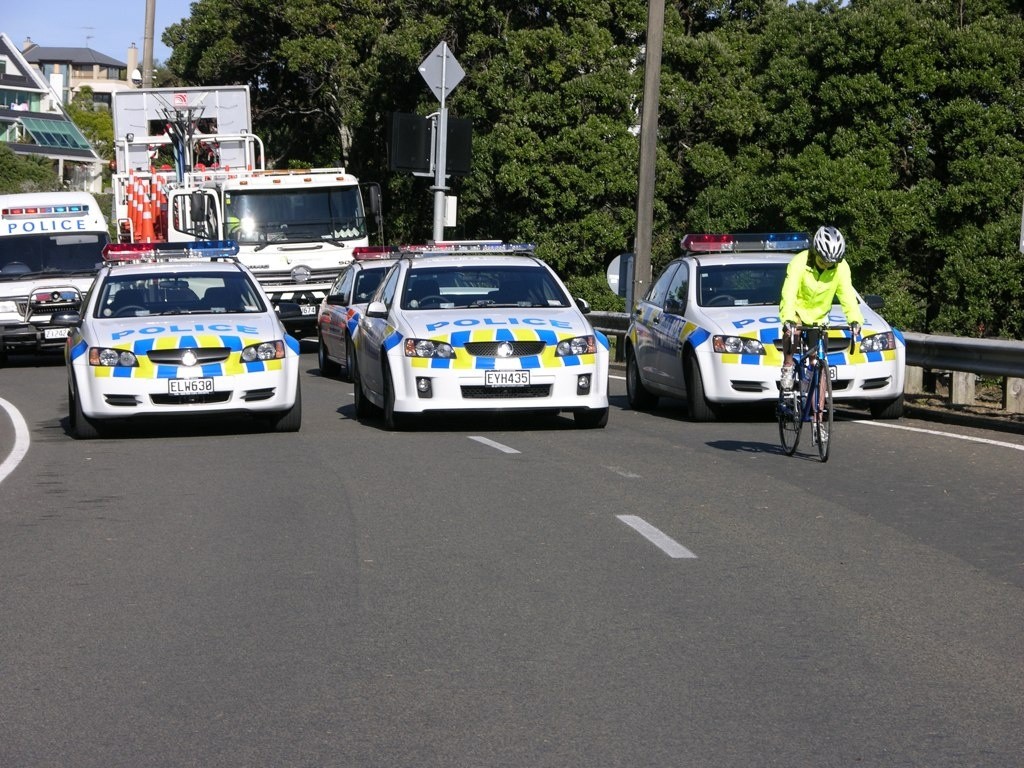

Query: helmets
left=813, top=226, right=846, bottom=263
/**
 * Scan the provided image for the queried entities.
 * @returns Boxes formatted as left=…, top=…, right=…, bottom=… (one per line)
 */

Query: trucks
left=110, top=85, right=384, bottom=323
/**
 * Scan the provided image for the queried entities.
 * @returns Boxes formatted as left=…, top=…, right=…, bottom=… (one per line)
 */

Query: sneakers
left=780, top=363, right=795, bottom=391
left=813, top=422, right=826, bottom=443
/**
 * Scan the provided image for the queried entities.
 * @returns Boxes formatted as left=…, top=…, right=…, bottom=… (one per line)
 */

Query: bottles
left=801, top=364, right=813, bottom=392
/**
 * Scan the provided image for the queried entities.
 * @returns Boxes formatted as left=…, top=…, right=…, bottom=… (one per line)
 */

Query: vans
left=0, top=191, right=113, bottom=369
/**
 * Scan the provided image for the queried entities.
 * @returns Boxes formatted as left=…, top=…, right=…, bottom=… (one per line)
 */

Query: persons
left=779, top=225, right=865, bottom=444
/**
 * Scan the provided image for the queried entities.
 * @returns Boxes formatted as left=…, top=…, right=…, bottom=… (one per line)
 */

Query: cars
left=50, top=240, right=302, bottom=441
left=316, top=245, right=500, bottom=384
left=623, top=232, right=906, bottom=422
left=352, top=243, right=610, bottom=431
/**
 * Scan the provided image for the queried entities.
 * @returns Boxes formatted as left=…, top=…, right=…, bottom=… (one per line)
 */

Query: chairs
left=201, top=287, right=233, bottom=303
left=111, top=289, right=146, bottom=314
left=407, top=279, right=442, bottom=305
left=702, top=270, right=726, bottom=303
left=488, top=280, right=519, bottom=304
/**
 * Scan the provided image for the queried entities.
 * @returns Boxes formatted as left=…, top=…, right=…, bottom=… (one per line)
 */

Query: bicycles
left=775, top=321, right=862, bottom=462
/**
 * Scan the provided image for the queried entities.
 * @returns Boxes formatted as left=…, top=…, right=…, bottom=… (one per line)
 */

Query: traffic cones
left=121, top=166, right=179, bottom=244
